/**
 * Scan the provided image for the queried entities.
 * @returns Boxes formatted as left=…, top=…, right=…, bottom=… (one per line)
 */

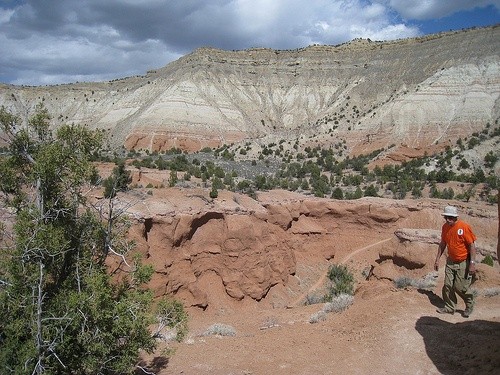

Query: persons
left=434, top=207, right=477, bottom=317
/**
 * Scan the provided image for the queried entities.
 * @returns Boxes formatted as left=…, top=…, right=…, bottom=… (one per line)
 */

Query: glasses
left=443, top=216, right=454, bottom=220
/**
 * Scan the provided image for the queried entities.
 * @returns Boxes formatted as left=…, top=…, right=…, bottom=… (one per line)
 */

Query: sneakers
left=461, top=307, right=474, bottom=317
left=436, top=308, right=455, bottom=314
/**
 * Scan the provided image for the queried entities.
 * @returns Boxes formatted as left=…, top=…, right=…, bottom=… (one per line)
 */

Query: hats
left=441, top=205, right=459, bottom=217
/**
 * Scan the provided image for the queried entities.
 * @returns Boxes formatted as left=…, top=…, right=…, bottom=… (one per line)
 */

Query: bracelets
left=470, top=261, right=475, bottom=265
